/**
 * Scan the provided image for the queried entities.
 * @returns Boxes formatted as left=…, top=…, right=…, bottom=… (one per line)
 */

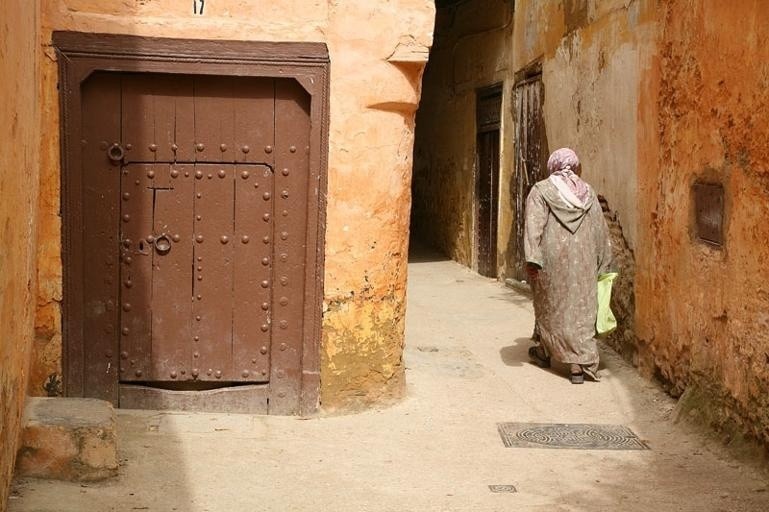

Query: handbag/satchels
left=593, top=267, right=619, bottom=337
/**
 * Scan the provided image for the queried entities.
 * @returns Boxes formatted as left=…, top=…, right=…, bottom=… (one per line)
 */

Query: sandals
left=528, top=346, right=551, bottom=369
left=568, top=366, right=585, bottom=384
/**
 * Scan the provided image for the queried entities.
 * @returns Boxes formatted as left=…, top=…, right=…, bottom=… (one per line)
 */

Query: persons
left=522, top=147, right=614, bottom=387
